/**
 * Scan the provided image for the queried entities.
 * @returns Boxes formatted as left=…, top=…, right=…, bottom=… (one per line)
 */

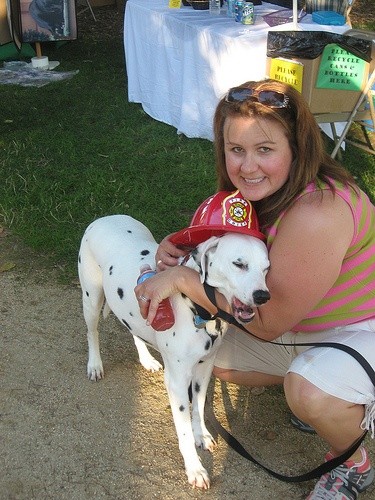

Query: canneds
left=227, top=0, right=255, bottom=25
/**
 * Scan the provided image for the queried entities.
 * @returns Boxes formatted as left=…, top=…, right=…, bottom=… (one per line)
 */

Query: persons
left=134, top=79, right=375, bottom=500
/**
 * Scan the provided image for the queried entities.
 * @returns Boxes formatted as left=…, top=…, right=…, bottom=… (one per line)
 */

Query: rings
left=156, top=260, right=162, bottom=265
left=141, top=295, right=147, bottom=302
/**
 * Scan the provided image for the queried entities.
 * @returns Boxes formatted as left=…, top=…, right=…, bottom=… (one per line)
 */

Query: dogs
left=76, top=214, right=269, bottom=491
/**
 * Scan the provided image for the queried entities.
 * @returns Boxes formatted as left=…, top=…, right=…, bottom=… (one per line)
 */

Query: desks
left=124, top=0, right=352, bottom=141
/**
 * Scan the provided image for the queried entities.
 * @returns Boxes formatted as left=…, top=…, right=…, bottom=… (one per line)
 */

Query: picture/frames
left=19, top=0, right=78, bottom=42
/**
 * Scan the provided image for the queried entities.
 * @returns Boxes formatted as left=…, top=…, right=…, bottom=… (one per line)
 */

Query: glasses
left=225, top=87, right=295, bottom=113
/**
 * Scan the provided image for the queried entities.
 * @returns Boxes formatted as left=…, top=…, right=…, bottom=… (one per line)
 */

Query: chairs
left=313, top=71, right=375, bottom=159
left=306, top=0, right=355, bottom=27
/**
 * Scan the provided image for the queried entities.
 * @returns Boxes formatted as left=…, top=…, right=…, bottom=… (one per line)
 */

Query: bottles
left=209, top=0, right=220, bottom=16
left=136, top=264, right=175, bottom=331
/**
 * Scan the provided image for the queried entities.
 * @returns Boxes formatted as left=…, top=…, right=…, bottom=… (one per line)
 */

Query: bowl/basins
left=263, top=11, right=306, bottom=27
left=186, top=0, right=210, bottom=10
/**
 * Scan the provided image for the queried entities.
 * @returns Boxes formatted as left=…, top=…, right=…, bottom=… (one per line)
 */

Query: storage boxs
left=266, top=30, right=375, bottom=113
left=312, top=11, right=347, bottom=26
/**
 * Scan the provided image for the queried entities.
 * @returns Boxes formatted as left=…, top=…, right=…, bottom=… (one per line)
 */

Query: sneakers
left=304, top=447, right=374, bottom=500
